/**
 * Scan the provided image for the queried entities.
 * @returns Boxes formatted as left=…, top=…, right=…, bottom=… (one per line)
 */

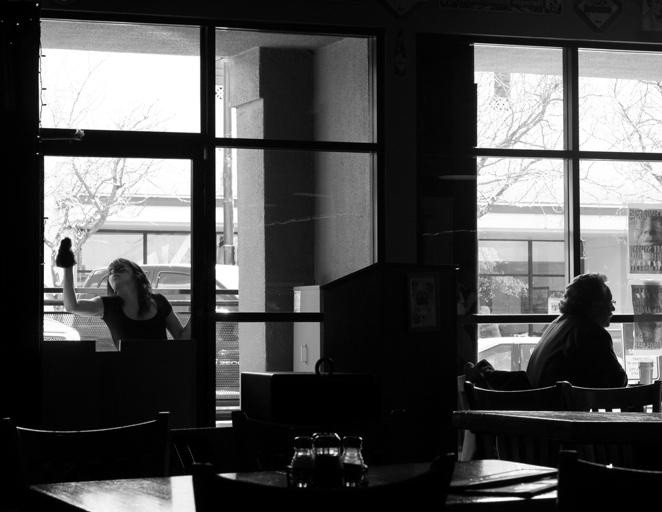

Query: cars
left=43, top=317, right=80, bottom=341
left=69, top=264, right=239, bottom=381
left=477, top=337, right=539, bottom=371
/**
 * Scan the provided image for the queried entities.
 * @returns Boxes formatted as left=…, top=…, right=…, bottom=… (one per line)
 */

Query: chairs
left=0, top=339, right=660, bottom=512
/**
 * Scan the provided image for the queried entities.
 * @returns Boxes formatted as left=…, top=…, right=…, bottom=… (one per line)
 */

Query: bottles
left=291, top=432, right=365, bottom=487
left=638, top=362, right=654, bottom=385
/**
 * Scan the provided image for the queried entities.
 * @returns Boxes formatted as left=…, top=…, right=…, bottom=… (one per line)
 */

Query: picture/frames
left=408, top=272, right=439, bottom=332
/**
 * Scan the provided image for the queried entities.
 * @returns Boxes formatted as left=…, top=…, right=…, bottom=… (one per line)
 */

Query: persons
left=56, top=238, right=192, bottom=350
left=525, top=273, right=628, bottom=389
left=629, top=210, right=662, bottom=247
